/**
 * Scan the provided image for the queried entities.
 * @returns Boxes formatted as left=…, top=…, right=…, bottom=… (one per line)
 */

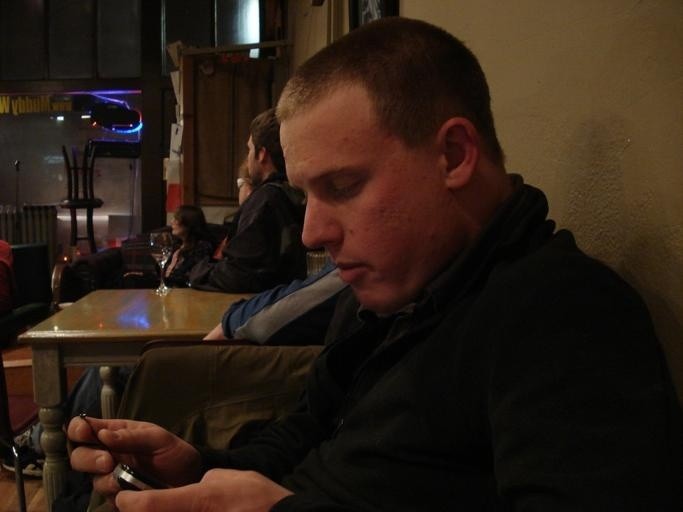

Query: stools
left=59, top=144, right=103, bottom=254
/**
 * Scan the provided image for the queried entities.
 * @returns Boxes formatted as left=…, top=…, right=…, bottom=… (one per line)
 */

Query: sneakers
left=2, top=451, right=45, bottom=476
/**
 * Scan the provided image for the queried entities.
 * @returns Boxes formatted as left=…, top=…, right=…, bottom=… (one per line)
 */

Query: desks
left=18, top=288, right=262, bottom=512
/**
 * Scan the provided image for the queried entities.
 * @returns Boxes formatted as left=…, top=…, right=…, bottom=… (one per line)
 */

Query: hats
left=0, top=239, right=14, bottom=269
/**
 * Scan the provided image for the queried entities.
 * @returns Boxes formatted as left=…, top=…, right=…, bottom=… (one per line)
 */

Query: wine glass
left=150, top=232, right=174, bottom=296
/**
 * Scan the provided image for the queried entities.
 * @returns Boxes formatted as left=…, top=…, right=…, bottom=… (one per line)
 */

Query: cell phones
left=112, top=462, right=170, bottom=492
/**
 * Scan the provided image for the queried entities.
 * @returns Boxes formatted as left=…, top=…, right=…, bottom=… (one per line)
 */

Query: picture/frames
left=348, top=0, right=399, bottom=31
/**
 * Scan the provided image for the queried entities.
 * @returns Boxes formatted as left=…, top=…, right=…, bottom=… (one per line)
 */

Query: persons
left=211, top=158, right=254, bottom=261
left=198, top=256, right=354, bottom=345
left=122, top=204, right=213, bottom=291
left=0, top=240, right=17, bottom=315
left=64, top=16, right=682, bottom=510
left=186, top=107, right=325, bottom=294
